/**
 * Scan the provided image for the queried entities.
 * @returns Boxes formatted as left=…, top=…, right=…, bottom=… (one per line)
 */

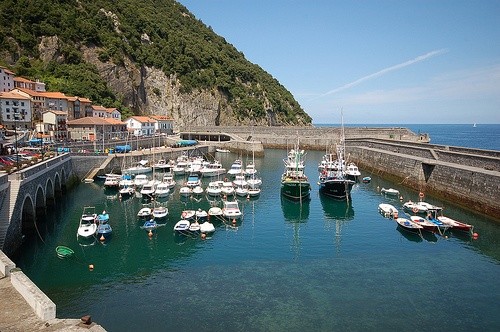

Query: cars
left=0, top=154, right=38, bottom=171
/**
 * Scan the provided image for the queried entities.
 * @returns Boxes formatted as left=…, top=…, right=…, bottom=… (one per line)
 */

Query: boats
left=75, top=173, right=119, bottom=244
left=363, top=177, right=372, bottom=183
left=381, top=188, right=399, bottom=196
left=56, top=246, right=74, bottom=259
left=174, top=153, right=263, bottom=238
left=396, top=215, right=475, bottom=233
left=137, top=206, right=169, bottom=233
left=378, top=203, right=399, bottom=219
left=403, top=201, right=443, bottom=213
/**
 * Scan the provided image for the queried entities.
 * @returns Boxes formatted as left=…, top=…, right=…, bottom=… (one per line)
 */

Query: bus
left=18, top=139, right=41, bottom=147
left=18, top=148, right=42, bottom=153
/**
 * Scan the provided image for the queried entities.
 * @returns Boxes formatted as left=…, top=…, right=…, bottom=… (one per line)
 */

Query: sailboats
left=282, top=129, right=312, bottom=202
left=320, top=106, right=361, bottom=201
left=120, top=125, right=176, bottom=200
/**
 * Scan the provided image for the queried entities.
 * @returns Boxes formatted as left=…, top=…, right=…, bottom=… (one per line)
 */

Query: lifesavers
left=249, top=161, right=253, bottom=165
left=413, top=206, right=419, bottom=213
left=181, top=212, right=187, bottom=216
left=419, top=192, right=424, bottom=198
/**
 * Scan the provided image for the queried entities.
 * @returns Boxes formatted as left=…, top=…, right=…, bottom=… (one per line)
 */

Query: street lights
left=12, top=99, right=23, bottom=172
